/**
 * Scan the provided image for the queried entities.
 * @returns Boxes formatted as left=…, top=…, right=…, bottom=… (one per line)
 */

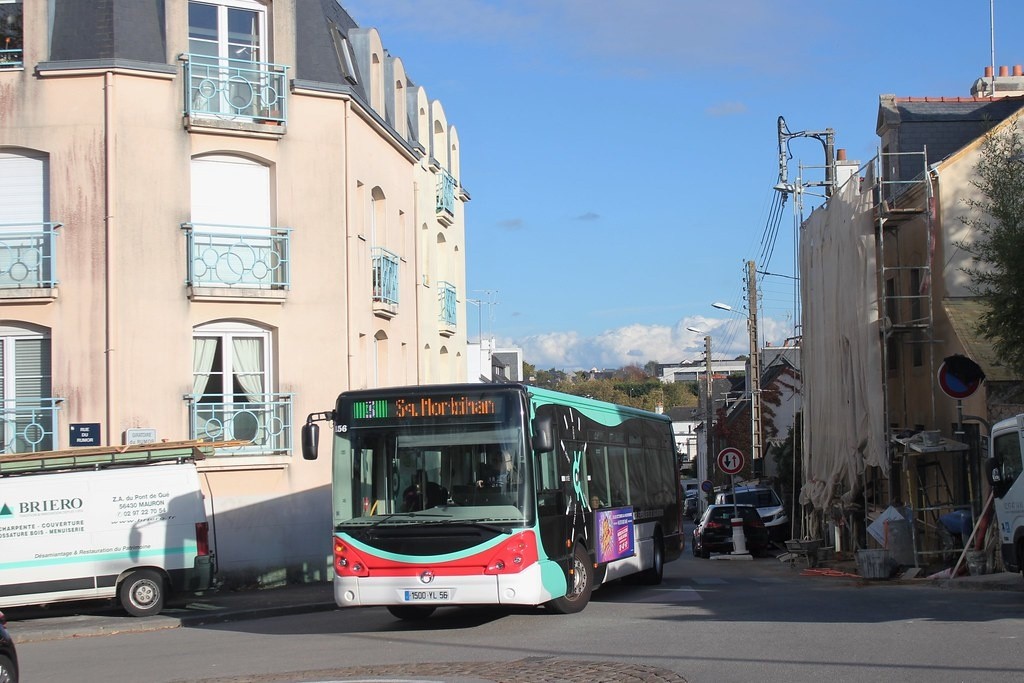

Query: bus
left=300, top=380, right=687, bottom=623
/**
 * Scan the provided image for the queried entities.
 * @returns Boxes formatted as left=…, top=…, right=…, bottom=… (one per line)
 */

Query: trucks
left=984, top=413, right=1024, bottom=576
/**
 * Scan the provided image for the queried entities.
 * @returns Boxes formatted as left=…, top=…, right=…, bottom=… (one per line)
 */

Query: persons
left=590, top=493, right=600, bottom=509
left=402, top=469, right=443, bottom=514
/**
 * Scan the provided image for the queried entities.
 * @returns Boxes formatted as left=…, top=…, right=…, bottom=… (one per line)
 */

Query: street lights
left=712, top=260, right=764, bottom=485
left=686, top=326, right=713, bottom=482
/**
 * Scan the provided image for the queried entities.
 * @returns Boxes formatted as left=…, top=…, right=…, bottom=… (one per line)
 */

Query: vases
left=261, top=109, right=279, bottom=125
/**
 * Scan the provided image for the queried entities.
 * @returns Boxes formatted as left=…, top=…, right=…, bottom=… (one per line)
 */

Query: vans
left=715, top=485, right=788, bottom=542
left=0, top=442, right=218, bottom=618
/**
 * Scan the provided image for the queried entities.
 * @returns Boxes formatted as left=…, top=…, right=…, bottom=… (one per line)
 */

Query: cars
left=692, top=503, right=768, bottom=559
left=683, top=489, right=698, bottom=516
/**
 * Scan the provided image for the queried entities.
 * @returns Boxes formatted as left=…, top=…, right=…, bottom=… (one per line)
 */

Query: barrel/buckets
left=882, top=519, right=917, bottom=565
left=857, top=549, right=889, bottom=579
left=966, top=550, right=987, bottom=575
